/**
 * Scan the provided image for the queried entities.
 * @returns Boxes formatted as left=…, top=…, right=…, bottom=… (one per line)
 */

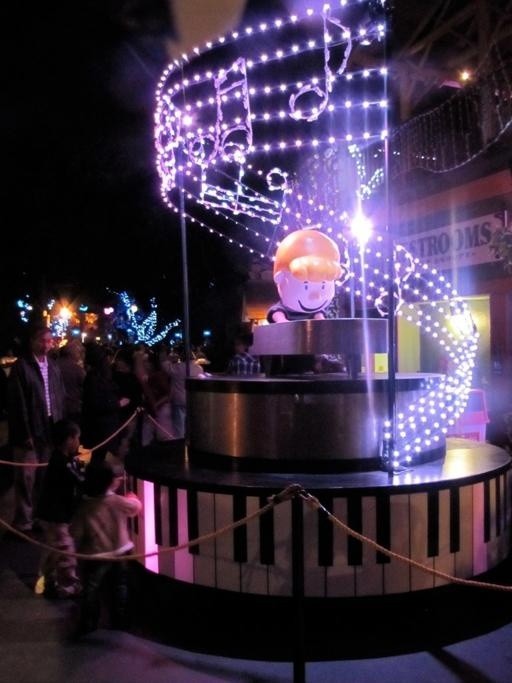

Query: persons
left=265, top=226, right=343, bottom=321
left=4, top=322, right=261, bottom=604
